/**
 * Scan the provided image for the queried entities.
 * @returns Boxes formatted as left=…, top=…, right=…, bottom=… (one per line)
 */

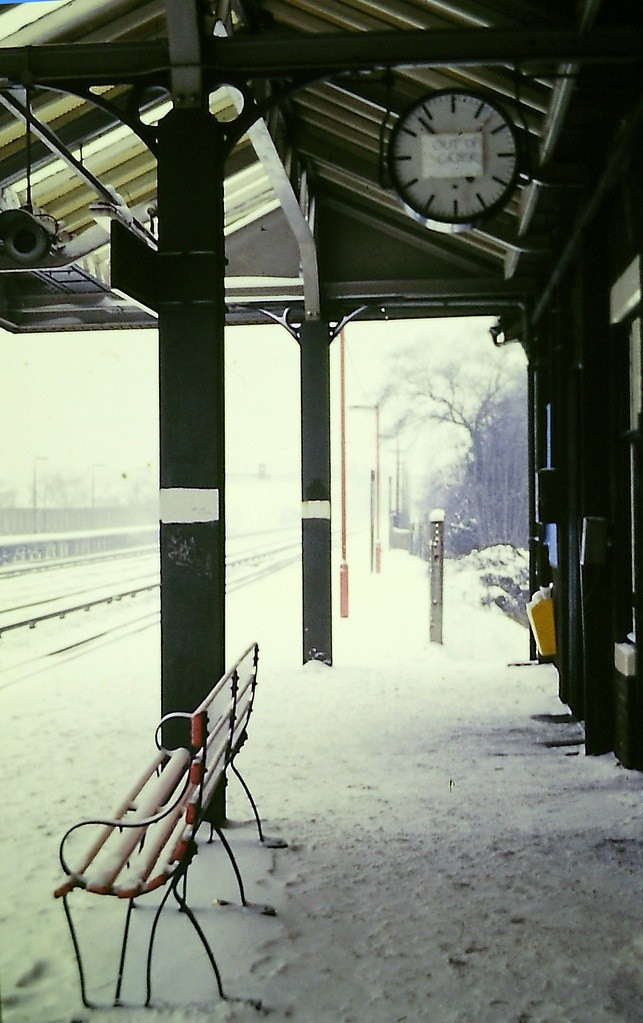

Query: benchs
left=48, top=640, right=288, bottom=1015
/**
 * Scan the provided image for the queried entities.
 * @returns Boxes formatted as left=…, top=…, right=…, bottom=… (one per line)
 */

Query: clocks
left=387, top=86, right=526, bottom=223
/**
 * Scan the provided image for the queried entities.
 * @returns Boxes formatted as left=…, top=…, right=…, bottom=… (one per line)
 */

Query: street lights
left=349, top=401, right=382, bottom=573
